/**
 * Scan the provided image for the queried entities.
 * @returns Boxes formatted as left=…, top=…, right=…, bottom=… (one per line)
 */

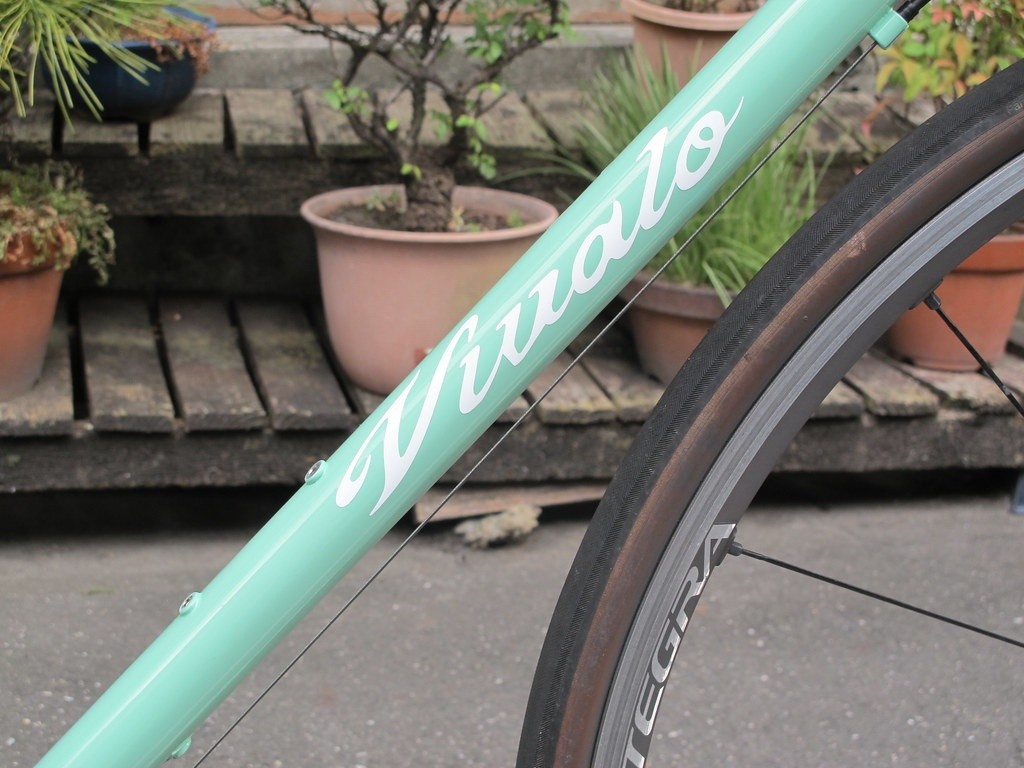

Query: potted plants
left=628, top=0, right=768, bottom=97
left=243, top=0, right=578, bottom=400
left=543, top=36, right=842, bottom=390
left=871, top=0, right=1024, bottom=374
left=1, top=145, right=115, bottom=406
left=1, top=0, right=216, bottom=135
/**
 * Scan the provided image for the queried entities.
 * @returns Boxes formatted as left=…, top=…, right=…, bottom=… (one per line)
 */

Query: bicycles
left=20, top=0, right=1023, bottom=768
